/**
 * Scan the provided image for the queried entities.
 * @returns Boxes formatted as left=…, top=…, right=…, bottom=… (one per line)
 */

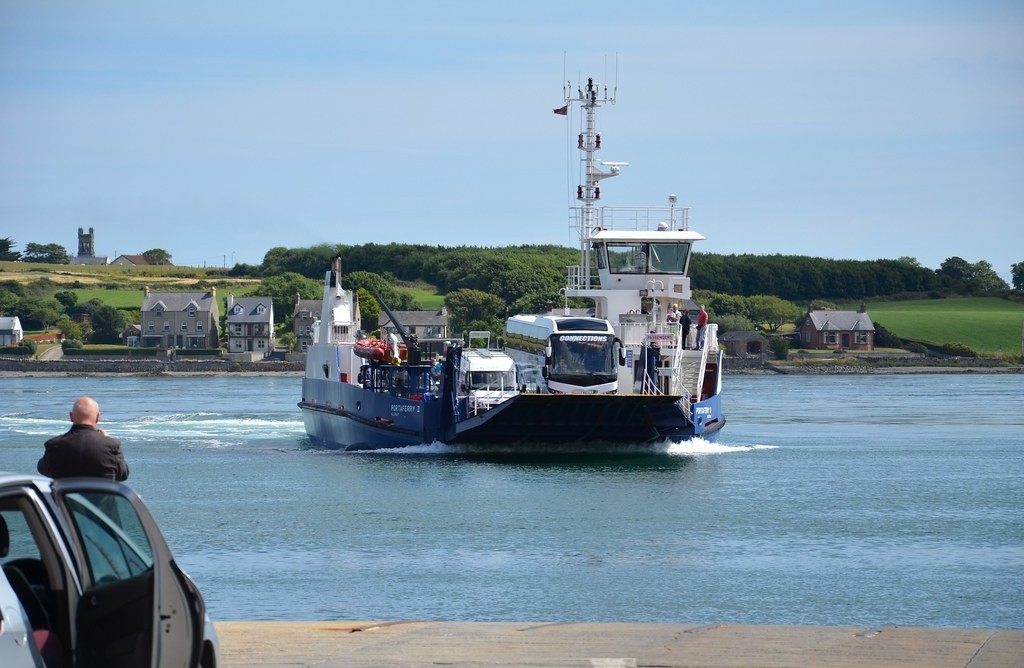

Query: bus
left=503, top=313, right=626, bottom=399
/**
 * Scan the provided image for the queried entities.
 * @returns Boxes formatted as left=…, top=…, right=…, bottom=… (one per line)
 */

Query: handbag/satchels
left=696, top=325, right=703, bottom=330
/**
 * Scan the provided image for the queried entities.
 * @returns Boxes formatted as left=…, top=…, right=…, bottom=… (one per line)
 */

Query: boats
left=296, top=54, right=727, bottom=453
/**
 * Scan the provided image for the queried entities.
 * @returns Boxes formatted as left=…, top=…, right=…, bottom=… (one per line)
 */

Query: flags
left=552, top=105, right=567, bottom=116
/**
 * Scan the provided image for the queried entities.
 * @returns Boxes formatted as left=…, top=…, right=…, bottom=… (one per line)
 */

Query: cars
left=0, top=470, right=221, bottom=668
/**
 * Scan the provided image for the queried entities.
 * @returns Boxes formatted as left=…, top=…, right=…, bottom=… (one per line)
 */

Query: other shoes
left=692, top=348, right=699, bottom=350
left=699, top=347, right=704, bottom=351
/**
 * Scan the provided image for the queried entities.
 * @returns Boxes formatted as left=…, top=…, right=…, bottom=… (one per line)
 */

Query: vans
left=458, top=347, right=526, bottom=410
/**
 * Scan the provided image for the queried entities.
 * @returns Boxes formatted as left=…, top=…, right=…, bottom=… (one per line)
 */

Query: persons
left=679, top=308, right=692, bottom=351
left=692, top=303, right=708, bottom=350
left=37, top=397, right=129, bottom=531
left=666, top=304, right=682, bottom=349
